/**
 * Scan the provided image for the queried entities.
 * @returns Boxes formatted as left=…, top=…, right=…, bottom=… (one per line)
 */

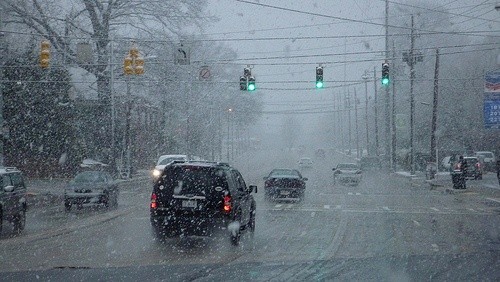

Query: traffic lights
left=315, top=66, right=323, bottom=89
left=381, top=63, right=389, bottom=85
left=247, top=76, right=256, bottom=91
left=240, top=76, right=247, bottom=90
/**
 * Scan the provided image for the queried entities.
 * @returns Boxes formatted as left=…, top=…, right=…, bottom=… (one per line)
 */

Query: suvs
left=0, top=166, right=28, bottom=232
left=149, top=159, right=259, bottom=245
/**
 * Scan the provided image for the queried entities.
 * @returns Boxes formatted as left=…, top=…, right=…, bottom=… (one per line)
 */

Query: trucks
left=152, top=154, right=188, bottom=180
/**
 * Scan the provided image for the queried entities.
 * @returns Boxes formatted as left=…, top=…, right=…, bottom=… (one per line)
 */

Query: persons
left=496, top=159, right=500, bottom=185
left=448, top=154, right=455, bottom=175
left=458, top=156, right=467, bottom=189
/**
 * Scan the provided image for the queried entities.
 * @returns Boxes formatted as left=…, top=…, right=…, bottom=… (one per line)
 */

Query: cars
left=314, top=149, right=325, bottom=157
left=332, top=163, right=364, bottom=185
left=65, top=171, right=119, bottom=212
left=297, top=158, right=313, bottom=168
left=441, top=151, right=496, bottom=175
left=262, top=169, right=308, bottom=200
left=404, top=152, right=438, bottom=171
